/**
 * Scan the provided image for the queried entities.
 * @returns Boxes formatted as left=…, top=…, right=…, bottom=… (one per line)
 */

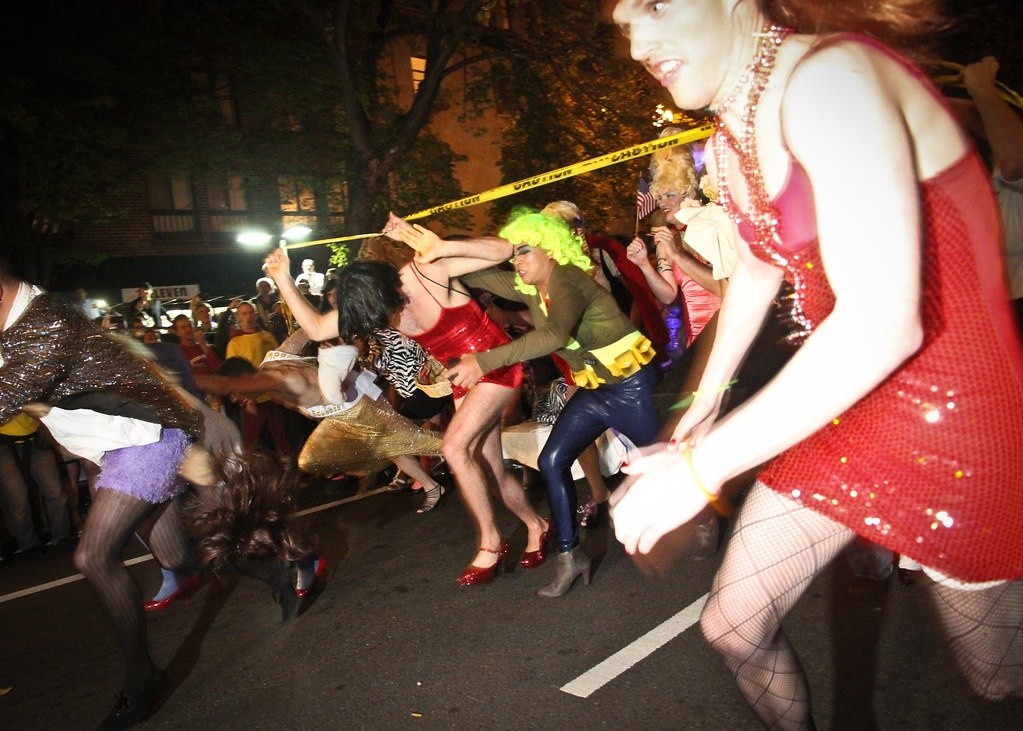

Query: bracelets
left=685, top=438, right=719, bottom=501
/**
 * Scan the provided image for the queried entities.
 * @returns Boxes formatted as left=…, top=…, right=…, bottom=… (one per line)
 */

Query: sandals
left=415, top=483, right=446, bottom=514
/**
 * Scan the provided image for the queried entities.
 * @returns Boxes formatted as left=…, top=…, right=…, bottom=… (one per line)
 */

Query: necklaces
left=712, top=21, right=813, bottom=345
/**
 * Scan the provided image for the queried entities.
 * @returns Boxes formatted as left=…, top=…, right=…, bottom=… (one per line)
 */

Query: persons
left=945, top=56, right=1023, bottom=343
left=0, top=126, right=740, bottom=731
left=843, top=539, right=895, bottom=596
left=598, top=0, right=1023, bottom=731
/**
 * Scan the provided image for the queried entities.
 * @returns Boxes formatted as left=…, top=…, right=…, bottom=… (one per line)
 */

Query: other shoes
left=577, top=493, right=614, bottom=528
left=408, top=479, right=423, bottom=493
left=387, top=476, right=406, bottom=490
left=110, top=672, right=167, bottom=723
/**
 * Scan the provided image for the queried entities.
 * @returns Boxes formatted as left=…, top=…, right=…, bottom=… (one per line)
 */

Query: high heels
left=143, top=573, right=200, bottom=610
left=519, top=515, right=556, bottom=567
left=289, top=555, right=328, bottom=602
left=458, top=539, right=510, bottom=586
left=536, top=547, right=592, bottom=598
